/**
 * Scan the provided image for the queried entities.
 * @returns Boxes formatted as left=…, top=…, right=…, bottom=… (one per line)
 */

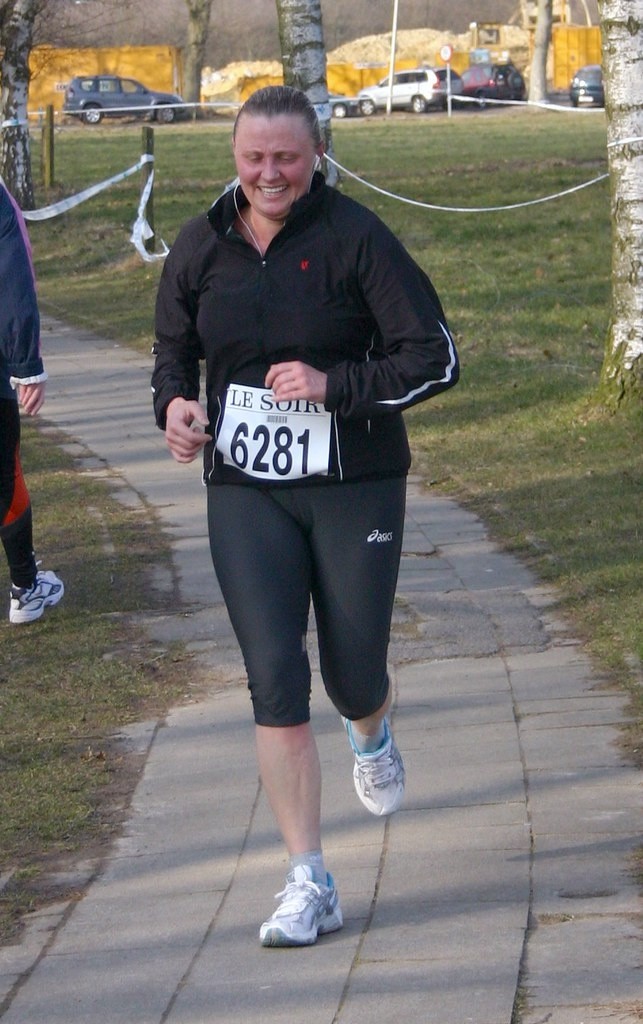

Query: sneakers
left=9, top=560, right=65, bottom=624
left=340, top=714, right=405, bottom=816
left=260, top=871, right=343, bottom=947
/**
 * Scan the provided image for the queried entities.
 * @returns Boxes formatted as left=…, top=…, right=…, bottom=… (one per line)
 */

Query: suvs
left=356, top=65, right=464, bottom=117
left=456, top=62, right=525, bottom=111
left=61, top=74, right=187, bottom=126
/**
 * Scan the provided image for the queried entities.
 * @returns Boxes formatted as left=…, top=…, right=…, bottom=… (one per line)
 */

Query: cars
left=570, top=64, right=605, bottom=108
left=325, top=92, right=361, bottom=120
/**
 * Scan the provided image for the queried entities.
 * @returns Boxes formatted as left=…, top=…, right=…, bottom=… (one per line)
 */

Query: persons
left=149, top=85, right=460, bottom=947
left=0, top=177, right=64, bottom=623
